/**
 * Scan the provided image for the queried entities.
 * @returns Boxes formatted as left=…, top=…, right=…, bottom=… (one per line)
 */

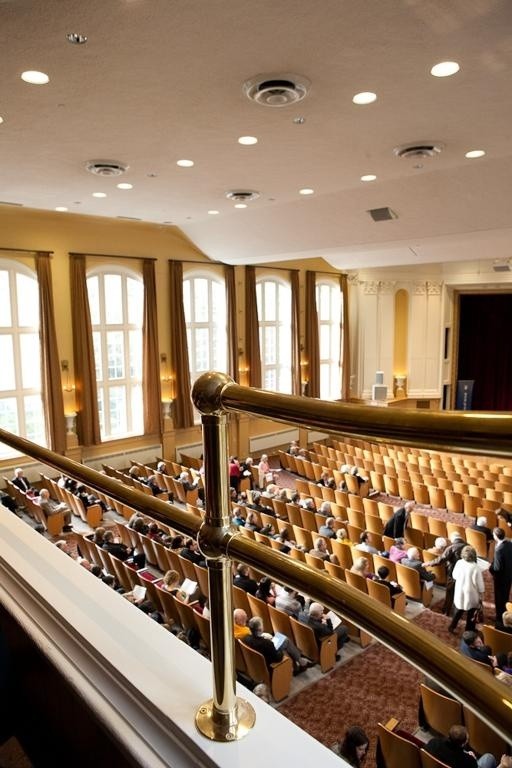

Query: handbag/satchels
left=471, top=608, right=484, bottom=624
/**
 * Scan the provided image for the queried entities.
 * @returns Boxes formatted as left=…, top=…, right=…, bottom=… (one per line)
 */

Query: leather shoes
left=336, top=654, right=341, bottom=662
left=293, top=662, right=316, bottom=676
left=345, top=636, right=351, bottom=643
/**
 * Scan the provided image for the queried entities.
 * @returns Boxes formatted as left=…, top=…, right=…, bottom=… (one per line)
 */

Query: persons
left=10, top=441, right=447, bottom=701
left=420, top=516, right=511, bottom=675
left=425, top=725, right=511, bottom=767
left=332, top=726, right=370, bottom=767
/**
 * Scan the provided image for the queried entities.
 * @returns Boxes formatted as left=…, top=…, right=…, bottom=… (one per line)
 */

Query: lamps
left=299, top=343, right=308, bottom=366
left=61, top=359, right=75, bottom=392
left=161, top=399, right=173, bottom=419
left=161, top=353, right=173, bottom=383
left=238, top=348, right=250, bottom=375
left=395, top=377, right=407, bottom=388
left=302, top=382, right=308, bottom=396
left=64, top=412, right=77, bottom=433
